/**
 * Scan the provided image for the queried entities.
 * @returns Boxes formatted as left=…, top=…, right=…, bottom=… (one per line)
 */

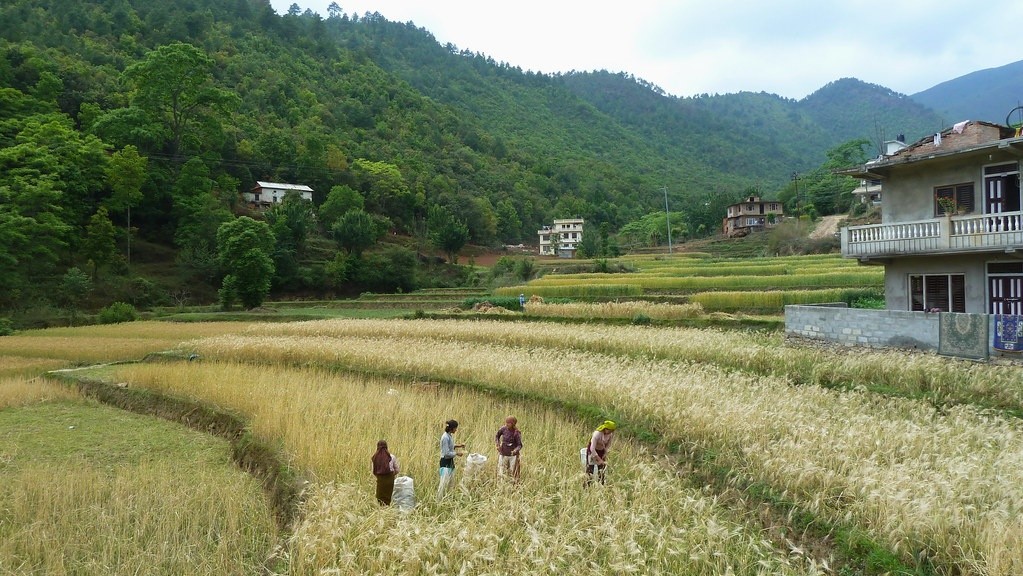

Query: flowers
left=938, top=196, right=956, bottom=211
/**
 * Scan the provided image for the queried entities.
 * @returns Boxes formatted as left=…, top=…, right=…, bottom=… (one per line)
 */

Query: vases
left=944, top=211, right=966, bottom=216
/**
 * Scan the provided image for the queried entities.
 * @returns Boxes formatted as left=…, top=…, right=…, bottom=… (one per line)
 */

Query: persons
left=583, top=421, right=616, bottom=488
left=371, top=440, right=400, bottom=506
left=495, top=415, right=522, bottom=473
left=437, top=420, right=465, bottom=496
left=520, top=293, right=524, bottom=306
